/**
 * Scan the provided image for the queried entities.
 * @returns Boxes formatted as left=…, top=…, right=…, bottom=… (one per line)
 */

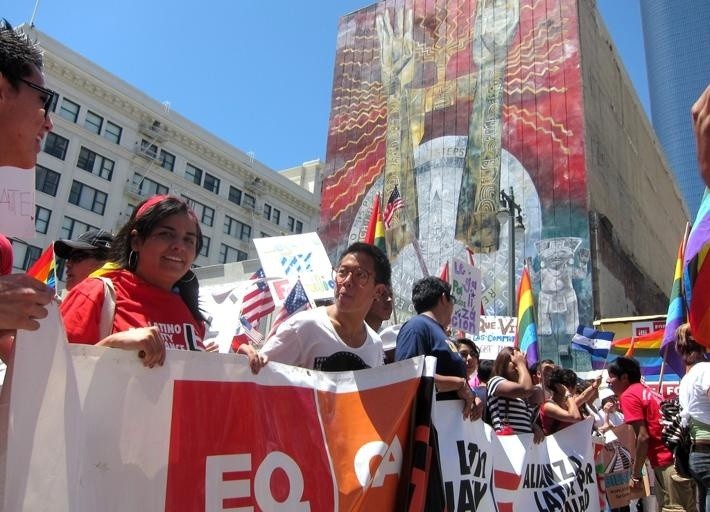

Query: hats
left=54, top=229, right=115, bottom=259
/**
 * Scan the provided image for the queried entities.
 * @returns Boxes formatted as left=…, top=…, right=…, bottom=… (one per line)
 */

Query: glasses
left=331, top=266, right=378, bottom=287
left=17, top=77, right=54, bottom=120
left=461, top=351, right=477, bottom=357
left=65, top=251, right=101, bottom=263
left=449, top=295, right=456, bottom=304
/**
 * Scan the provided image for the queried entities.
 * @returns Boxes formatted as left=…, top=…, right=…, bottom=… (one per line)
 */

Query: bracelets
left=591, top=383, right=601, bottom=390
left=630, top=475, right=645, bottom=483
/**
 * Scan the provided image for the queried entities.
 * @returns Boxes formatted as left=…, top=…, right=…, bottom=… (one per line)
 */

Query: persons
left=53, top=231, right=120, bottom=293
left=58, top=196, right=269, bottom=376
left=539, top=369, right=603, bottom=437
left=531, top=363, right=543, bottom=387
left=365, top=283, right=395, bottom=333
left=605, top=357, right=697, bottom=512
left=454, top=0, right=518, bottom=254
left=597, top=387, right=626, bottom=431
left=580, top=377, right=605, bottom=430
left=659, top=322, right=710, bottom=512
left=258, top=242, right=389, bottom=375
left=524, top=241, right=590, bottom=357
left=453, top=339, right=487, bottom=398
left=478, top=358, right=495, bottom=390
left=0, top=18, right=55, bottom=372
left=380, top=325, right=400, bottom=367
left=487, top=348, right=544, bottom=446
left=393, top=275, right=485, bottom=512
left=377, top=4, right=417, bottom=246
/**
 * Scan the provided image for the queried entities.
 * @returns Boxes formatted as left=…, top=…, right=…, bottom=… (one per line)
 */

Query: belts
left=689, top=443, right=709, bottom=453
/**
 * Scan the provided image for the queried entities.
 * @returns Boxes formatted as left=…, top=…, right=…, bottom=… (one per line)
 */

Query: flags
left=0, top=298, right=438, bottom=511
left=23, top=240, right=58, bottom=286
left=659, top=223, right=692, bottom=379
left=516, top=265, right=539, bottom=373
left=383, top=186, right=407, bottom=227
left=425, top=396, right=601, bottom=512
left=682, top=189, right=710, bottom=356
left=366, top=193, right=388, bottom=261
left=269, top=280, right=314, bottom=336
left=240, top=267, right=275, bottom=324
left=440, top=261, right=449, bottom=284
left=605, top=330, right=682, bottom=384
left=572, top=325, right=615, bottom=370
left=48, top=241, right=56, bottom=291
